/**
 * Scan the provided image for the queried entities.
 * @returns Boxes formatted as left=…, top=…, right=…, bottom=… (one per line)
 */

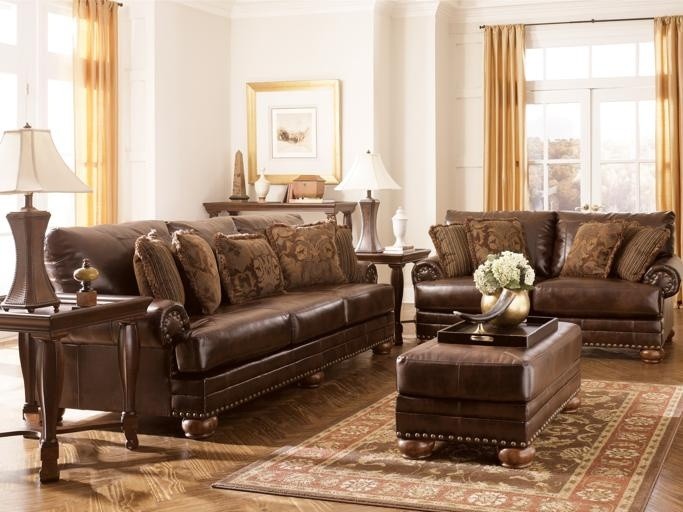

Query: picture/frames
left=246, top=80, right=342, bottom=184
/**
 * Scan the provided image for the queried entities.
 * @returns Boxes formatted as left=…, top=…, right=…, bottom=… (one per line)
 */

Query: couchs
left=19, top=214, right=395, bottom=439
left=411, top=209, right=683, bottom=364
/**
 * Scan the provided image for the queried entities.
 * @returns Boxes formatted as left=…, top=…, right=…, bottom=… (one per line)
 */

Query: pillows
left=557, top=221, right=670, bottom=282
left=429, top=218, right=535, bottom=279
left=133, top=219, right=364, bottom=314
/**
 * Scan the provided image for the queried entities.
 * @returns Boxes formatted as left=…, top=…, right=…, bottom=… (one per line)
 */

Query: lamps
left=334, top=149, right=401, bottom=253
left=1, top=123, right=93, bottom=313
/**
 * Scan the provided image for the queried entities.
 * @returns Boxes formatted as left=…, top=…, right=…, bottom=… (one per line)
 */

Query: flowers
left=473, top=251, right=536, bottom=295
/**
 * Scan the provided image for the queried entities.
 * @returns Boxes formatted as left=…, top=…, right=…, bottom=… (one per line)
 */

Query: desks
left=0, top=293, right=154, bottom=484
left=202, top=201, right=358, bottom=241
left=353, top=249, right=431, bottom=346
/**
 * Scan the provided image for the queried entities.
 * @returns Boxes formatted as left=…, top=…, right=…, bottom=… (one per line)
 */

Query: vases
left=481, top=289, right=530, bottom=330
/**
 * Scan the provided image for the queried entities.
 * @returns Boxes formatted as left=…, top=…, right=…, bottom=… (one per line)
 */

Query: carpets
left=212, top=378, right=683, bottom=512
